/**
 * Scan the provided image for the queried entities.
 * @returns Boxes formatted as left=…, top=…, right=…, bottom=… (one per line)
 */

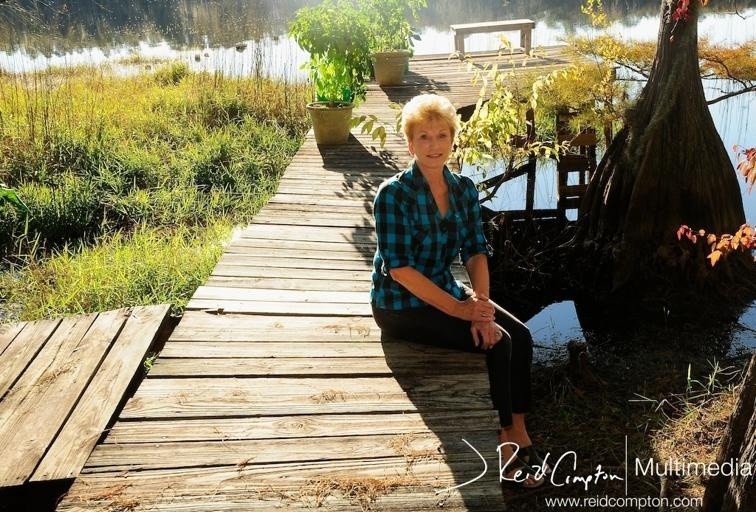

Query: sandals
left=513, top=445, right=552, bottom=477
left=502, top=458, right=545, bottom=489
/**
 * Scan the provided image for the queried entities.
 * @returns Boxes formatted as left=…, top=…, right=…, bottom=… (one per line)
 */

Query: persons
left=370, top=93, right=562, bottom=485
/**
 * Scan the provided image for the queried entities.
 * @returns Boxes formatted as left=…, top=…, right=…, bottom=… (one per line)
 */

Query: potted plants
left=287, top=0, right=430, bottom=147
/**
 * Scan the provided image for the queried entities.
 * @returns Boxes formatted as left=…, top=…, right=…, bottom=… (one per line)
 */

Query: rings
left=481, top=311, right=493, bottom=320
left=495, top=328, right=505, bottom=337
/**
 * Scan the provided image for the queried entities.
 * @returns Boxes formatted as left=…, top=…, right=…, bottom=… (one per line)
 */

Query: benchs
left=451, top=18, right=535, bottom=60
